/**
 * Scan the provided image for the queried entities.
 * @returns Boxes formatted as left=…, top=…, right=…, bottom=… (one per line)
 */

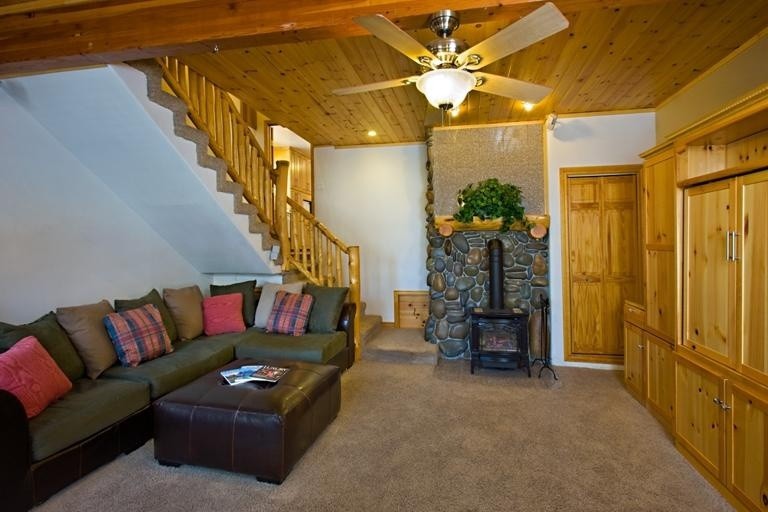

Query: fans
left=332, top=1, right=570, bottom=105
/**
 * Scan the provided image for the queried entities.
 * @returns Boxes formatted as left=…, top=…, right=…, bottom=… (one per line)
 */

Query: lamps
left=404, top=64, right=486, bottom=122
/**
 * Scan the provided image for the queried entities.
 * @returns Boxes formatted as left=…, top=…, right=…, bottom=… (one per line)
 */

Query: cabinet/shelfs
left=623, top=90, right=766, bottom=512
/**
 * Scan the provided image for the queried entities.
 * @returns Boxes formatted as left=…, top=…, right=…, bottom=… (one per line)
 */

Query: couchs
left=151, top=356, right=341, bottom=485
left=0, top=277, right=364, bottom=512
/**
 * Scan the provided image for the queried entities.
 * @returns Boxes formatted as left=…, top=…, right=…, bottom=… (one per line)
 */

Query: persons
left=484, top=336, right=513, bottom=349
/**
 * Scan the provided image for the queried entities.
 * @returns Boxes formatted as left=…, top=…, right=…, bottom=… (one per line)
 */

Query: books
left=219, top=364, right=293, bottom=387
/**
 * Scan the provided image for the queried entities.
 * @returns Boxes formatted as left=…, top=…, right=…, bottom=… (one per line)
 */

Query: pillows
left=253, top=282, right=304, bottom=331
left=162, top=285, right=205, bottom=343
left=114, top=287, right=180, bottom=349
left=1, top=334, right=74, bottom=419
left=303, top=280, right=350, bottom=334
left=210, top=279, right=258, bottom=331
left=203, top=292, right=246, bottom=337
left=265, top=290, right=314, bottom=338
left=1, top=309, right=86, bottom=384
left=101, top=302, right=176, bottom=370
left=55, top=298, right=119, bottom=381
